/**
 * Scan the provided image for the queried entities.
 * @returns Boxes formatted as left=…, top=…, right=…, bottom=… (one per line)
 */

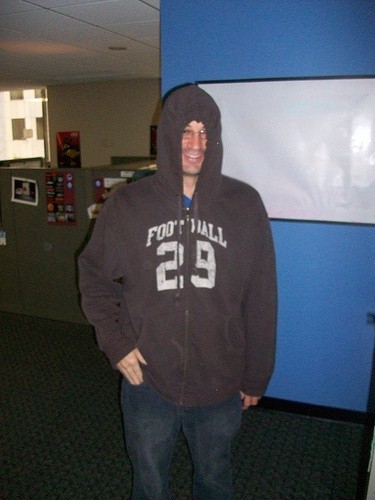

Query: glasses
left=181, top=128, right=207, bottom=140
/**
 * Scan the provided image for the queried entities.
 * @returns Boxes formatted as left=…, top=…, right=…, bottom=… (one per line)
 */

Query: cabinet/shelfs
left=87, top=165, right=156, bottom=244
left=17, top=167, right=87, bottom=323
left=1, top=166, right=20, bottom=314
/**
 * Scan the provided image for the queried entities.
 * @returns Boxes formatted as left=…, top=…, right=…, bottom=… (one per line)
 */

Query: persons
left=79, top=84, right=279, bottom=500
